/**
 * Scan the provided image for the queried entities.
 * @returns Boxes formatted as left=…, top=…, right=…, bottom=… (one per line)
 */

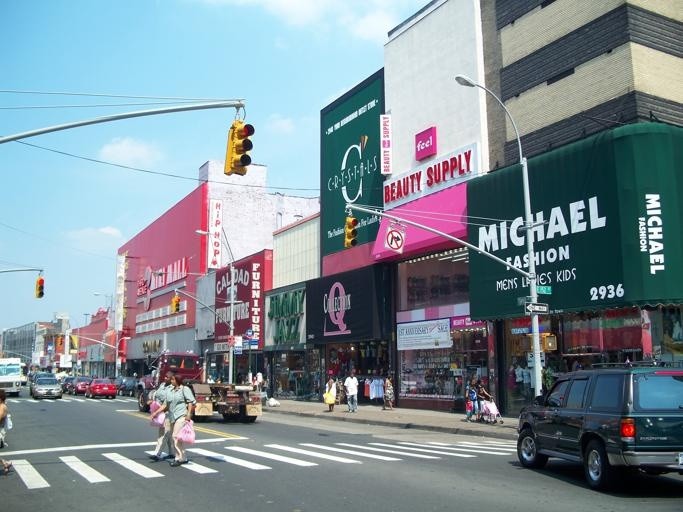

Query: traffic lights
left=171, top=295, right=181, bottom=314
left=35, top=277, right=46, bottom=298
left=342, top=216, right=359, bottom=249
left=223, top=119, right=255, bottom=179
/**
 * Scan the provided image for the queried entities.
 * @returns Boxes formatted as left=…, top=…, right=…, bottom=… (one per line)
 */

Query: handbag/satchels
left=323, top=392, right=336, bottom=405
left=5, top=413, right=14, bottom=430
left=174, top=419, right=195, bottom=444
left=148, top=401, right=166, bottom=428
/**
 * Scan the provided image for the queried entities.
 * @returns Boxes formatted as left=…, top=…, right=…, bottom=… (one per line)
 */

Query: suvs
left=511, top=358, right=683, bottom=495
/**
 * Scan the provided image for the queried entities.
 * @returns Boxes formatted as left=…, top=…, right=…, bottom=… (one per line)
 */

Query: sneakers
left=148, top=454, right=189, bottom=467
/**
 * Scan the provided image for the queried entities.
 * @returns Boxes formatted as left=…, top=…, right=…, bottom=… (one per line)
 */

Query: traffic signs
left=524, top=301, right=550, bottom=317
left=540, top=332, right=558, bottom=353
left=535, top=285, right=554, bottom=296
left=510, top=327, right=530, bottom=335
left=517, top=333, right=533, bottom=352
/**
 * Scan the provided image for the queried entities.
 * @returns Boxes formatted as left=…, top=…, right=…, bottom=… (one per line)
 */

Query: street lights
left=93, top=291, right=112, bottom=310
left=115, top=336, right=133, bottom=377
left=194, top=227, right=237, bottom=386
left=452, top=72, right=546, bottom=403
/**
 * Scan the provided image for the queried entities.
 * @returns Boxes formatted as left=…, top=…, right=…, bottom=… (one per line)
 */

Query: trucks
left=0, top=357, right=23, bottom=398
left=132, top=349, right=264, bottom=427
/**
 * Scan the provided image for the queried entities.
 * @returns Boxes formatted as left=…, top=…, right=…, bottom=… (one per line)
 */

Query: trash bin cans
left=260, top=392, right=267, bottom=406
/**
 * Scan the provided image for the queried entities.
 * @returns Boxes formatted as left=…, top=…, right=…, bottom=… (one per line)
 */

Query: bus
left=21, top=362, right=30, bottom=387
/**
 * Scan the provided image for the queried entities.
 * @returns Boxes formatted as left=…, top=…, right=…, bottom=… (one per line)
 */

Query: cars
left=276, top=364, right=367, bottom=403
left=26, top=370, right=145, bottom=401
left=559, top=345, right=610, bottom=367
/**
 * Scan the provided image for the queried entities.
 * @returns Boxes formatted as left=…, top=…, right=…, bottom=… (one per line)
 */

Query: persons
left=0, top=389, right=12, bottom=476
left=521, top=366, right=530, bottom=396
left=322, top=376, right=336, bottom=412
left=250, top=376, right=258, bottom=392
left=465, top=397, right=473, bottom=423
left=475, top=379, right=494, bottom=422
left=343, top=371, right=359, bottom=413
left=529, top=366, right=534, bottom=398
left=216, top=376, right=223, bottom=383
left=149, top=374, right=196, bottom=467
left=382, top=375, right=394, bottom=410
left=506, top=365, right=514, bottom=391
left=206, top=375, right=214, bottom=383
left=147, top=371, right=176, bottom=461
left=514, top=364, right=522, bottom=385
left=464, top=378, right=478, bottom=422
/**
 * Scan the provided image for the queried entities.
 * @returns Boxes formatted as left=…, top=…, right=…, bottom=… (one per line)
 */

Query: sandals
left=4, top=461, right=12, bottom=475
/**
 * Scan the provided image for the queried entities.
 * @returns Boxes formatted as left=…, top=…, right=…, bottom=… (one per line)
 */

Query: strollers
left=479, top=395, right=505, bottom=426
